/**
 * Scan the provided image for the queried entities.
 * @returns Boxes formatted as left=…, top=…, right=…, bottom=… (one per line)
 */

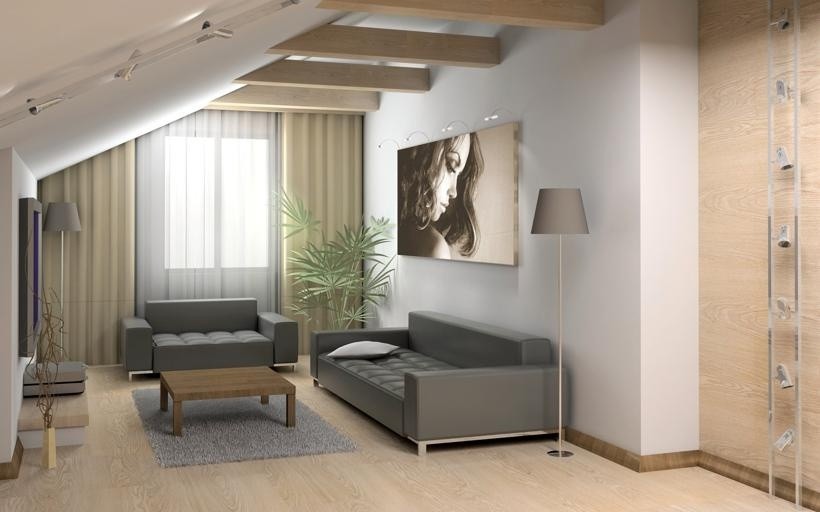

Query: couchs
left=119, top=299, right=298, bottom=380
left=310, top=311, right=572, bottom=456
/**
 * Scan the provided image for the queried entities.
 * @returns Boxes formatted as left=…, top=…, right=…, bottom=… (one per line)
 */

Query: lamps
left=43, top=203, right=85, bottom=359
left=369, top=109, right=516, bottom=153
left=26, top=91, right=65, bottom=116
left=197, top=21, right=233, bottom=43
left=765, top=1, right=801, bottom=509
left=529, top=188, right=590, bottom=458
left=114, top=49, right=143, bottom=79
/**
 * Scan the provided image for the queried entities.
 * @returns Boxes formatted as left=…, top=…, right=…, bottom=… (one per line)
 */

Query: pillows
left=324, top=339, right=400, bottom=358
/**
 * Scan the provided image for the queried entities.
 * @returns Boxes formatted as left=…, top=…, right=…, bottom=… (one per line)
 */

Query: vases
left=42, top=425, right=60, bottom=473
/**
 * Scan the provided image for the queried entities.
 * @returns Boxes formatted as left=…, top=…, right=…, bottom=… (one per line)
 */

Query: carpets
left=132, top=387, right=360, bottom=467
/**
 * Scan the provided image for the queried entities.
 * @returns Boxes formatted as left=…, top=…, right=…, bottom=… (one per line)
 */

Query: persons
left=396, top=130, right=484, bottom=261
